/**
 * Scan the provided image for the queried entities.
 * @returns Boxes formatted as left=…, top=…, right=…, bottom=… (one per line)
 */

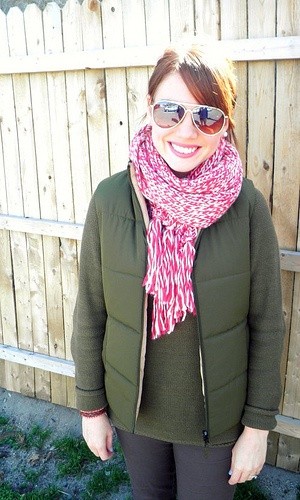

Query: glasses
left=148, top=101, right=230, bottom=136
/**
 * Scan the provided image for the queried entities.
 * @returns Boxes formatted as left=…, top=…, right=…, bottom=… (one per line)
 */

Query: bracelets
left=77, top=405, right=109, bottom=418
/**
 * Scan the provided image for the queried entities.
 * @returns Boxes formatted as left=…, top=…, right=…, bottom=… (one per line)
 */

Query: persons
left=70, top=38, right=283, bottom=500
left=197, top=107, right=209, bottom=125
left=176, top=105, right=184, bottom=120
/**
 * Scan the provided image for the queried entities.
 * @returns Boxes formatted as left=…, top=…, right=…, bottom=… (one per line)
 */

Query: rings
left=250, top=475, right=258, bottom=479
